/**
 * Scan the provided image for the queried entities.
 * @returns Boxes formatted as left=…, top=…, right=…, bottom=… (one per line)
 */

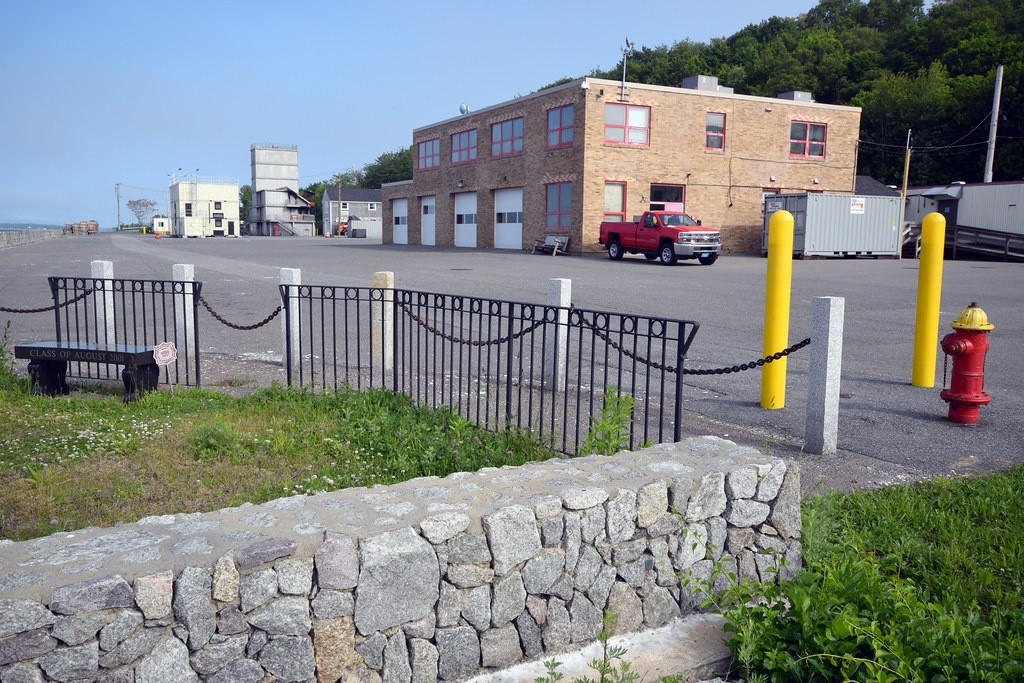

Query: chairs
left=668, top=218, right=675, bottom=224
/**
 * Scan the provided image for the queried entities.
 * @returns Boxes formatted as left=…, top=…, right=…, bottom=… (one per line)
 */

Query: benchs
left=532, top=234, right=570, bottom=256
left=14, top=341, right=161, bottom=402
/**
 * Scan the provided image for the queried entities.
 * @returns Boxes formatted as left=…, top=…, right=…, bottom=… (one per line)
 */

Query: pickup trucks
left=597, top=211, right=722, bottom=266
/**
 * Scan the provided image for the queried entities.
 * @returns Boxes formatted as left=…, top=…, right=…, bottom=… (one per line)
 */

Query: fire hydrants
left=940, top=301, right=992, bottom=425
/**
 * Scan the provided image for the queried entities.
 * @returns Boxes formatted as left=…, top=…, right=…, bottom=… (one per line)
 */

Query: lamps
left=458, top=180, right=463, bottom=188
